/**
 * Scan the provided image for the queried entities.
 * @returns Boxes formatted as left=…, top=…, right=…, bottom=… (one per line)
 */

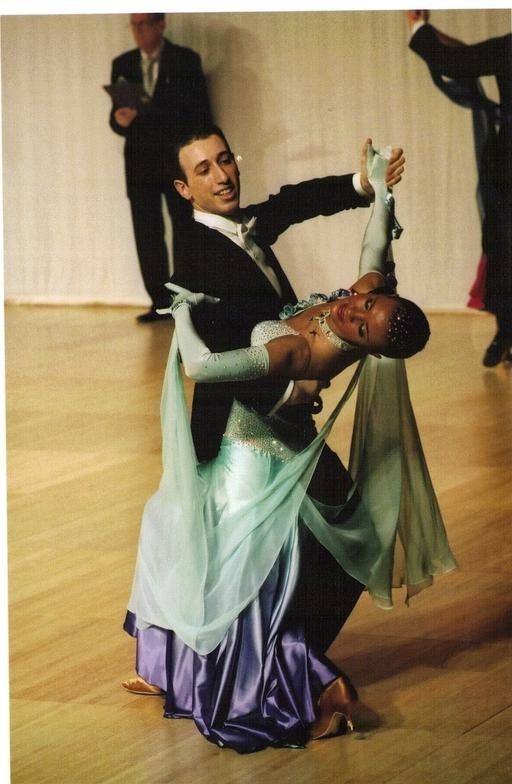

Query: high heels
left=306, top=672, right=378, bottom=743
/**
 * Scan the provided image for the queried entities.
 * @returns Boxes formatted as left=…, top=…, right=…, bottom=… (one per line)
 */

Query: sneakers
left=136, top=310, right=173, bottom=323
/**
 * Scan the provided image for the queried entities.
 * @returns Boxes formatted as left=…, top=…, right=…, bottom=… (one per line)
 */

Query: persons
left=407, top=10, right=502, bottom=314
left=100, top=13, right=216, bottom=325
left=119, top=139, right=458, bottom=756
left=403, top=9, right=512, bottom=369
left=159, top=116, right=409, bottom=659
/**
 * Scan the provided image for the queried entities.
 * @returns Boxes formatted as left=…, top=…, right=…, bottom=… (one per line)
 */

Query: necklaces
left=305, top=310, right=358, bottom=351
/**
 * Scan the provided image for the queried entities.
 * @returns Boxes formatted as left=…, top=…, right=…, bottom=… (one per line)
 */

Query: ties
left=141, top=54, right=159, bottom=97
left=235, top=217, right=267, bottom=263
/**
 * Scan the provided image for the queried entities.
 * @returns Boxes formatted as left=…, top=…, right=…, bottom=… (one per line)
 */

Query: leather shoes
left=483, top=333, right=508, bottom=369
left=118, top=675, right=163, bottom=696
